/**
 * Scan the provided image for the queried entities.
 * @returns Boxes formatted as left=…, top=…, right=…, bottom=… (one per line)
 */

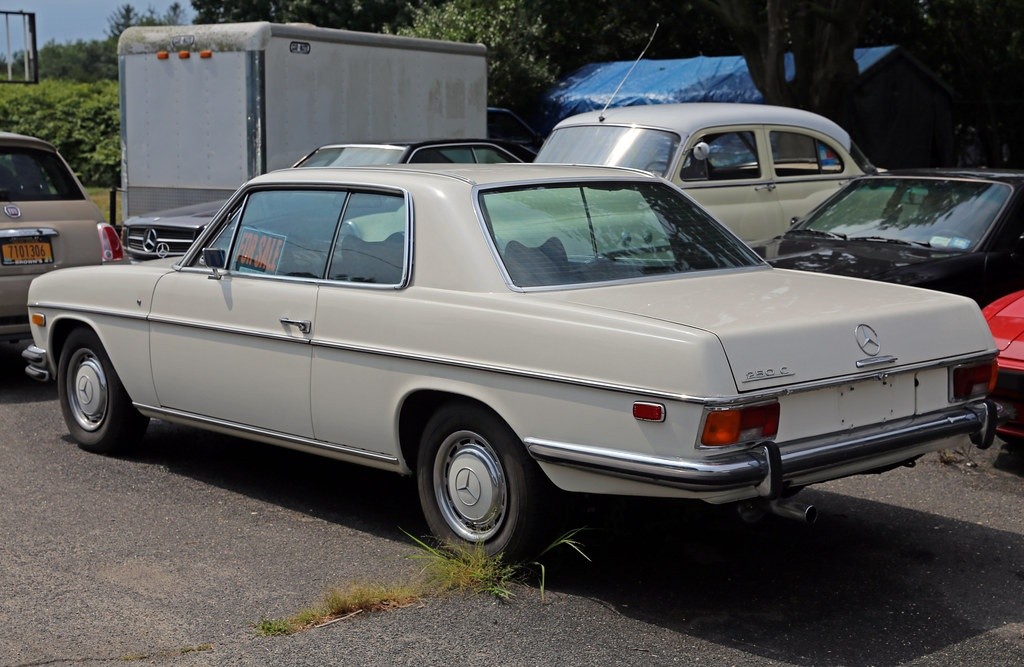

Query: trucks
left=116, top=21, right=545, bottom=217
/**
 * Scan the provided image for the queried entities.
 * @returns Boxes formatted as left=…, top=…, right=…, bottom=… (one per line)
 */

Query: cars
left=1, top=131, right=133, bottom=346
left=21, top=163, right=1001, bottom=568
left=980, top=290, right=1024, bottom=445
left=749, top=168, right=1024, bottom=312
left=340, top=102, right=961, bottom=277
left=121, top=137, right=539, bottom=263
